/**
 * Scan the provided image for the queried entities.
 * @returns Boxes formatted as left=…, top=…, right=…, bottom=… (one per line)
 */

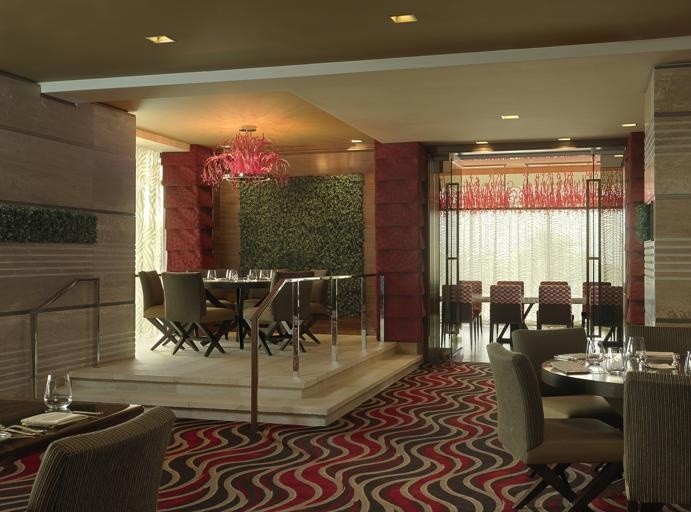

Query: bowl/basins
left=313, top=269, right=328, bottom=277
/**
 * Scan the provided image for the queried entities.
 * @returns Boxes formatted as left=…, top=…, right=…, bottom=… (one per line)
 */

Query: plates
left=0, top=431, right=11, bottom=442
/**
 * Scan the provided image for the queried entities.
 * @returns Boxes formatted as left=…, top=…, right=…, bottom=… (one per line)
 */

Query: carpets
left=0, top=359, right=691, bottom=511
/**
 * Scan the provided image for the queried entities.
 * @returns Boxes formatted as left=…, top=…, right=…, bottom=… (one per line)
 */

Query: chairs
left=625, top=324, right=690, bottom=361
left=623, top=372, right=690, bottom=512
left=512, top=327, right=623, bottom=432
left=441, top=280, right=625, bottom=348
left=486, top=343, right=626, bottom=512
left=24, top=405, right=178, bottom=511
left=139, top=267, right=331, bottom=356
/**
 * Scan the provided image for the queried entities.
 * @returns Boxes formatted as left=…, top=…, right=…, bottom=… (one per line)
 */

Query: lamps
left=198, top=125, right=292, bottom=197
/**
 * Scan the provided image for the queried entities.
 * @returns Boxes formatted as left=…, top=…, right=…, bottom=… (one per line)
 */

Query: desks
left=0, top=399, right=144, bottom=467
left=541, top=351, right=691, bottom=398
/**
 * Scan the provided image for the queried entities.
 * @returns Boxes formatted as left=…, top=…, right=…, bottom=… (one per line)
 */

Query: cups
left=585, top=334, right=691, bottom=377
left=42, top=371, right=74, bottom=410
left=206, top=269, right=277, bottom=282
